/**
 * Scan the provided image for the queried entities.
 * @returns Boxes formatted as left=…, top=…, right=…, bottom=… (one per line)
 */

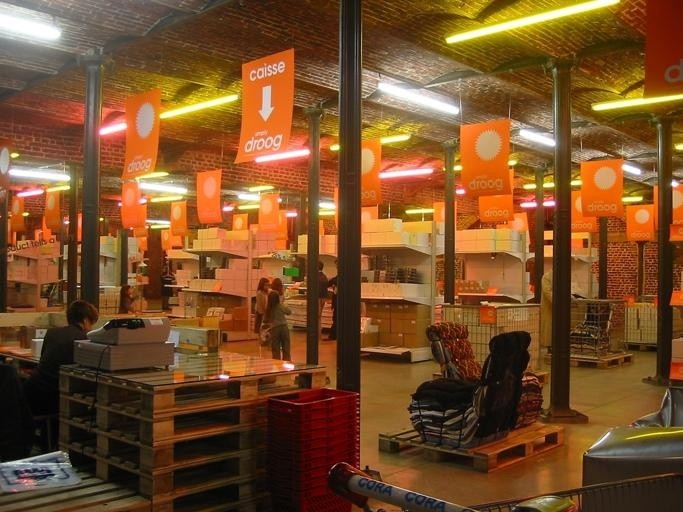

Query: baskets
left=268, top=388, right=361, bottom=512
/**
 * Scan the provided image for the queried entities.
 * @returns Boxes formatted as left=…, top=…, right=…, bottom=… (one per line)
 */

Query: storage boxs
left=265, top=387, right=360, bottom=511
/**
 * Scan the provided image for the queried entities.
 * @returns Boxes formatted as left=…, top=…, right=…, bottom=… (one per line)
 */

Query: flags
left=642, top=2, right=683, bottom=98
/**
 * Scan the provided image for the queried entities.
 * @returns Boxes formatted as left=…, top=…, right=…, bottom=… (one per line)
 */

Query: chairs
left=570, top=302, right=613, bottom=355
left=1, top=356, right=51, bottom=464
left=409, top=322, right=544, bottom=449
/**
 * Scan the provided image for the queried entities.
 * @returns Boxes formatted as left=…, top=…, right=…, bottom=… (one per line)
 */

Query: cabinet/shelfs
left=0, top=221, right=602, bottom=366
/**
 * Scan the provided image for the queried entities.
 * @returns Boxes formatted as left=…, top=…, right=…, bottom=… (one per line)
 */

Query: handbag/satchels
left=260, top=329, right=272, bottom=346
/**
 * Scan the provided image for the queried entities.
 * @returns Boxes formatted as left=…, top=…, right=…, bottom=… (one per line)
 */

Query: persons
left=268, top=276, right=285, bottom=304
left=313, top=259, right=328, bottom=339
left=116, top=283, right=141, bottom=315
left=22, top=298, right=100, bottom=448
left=260, top=291, right=293, bottom=363
left=321, top=259, right=337, bottom=341
left=252, top=277, right=271, bottom=333
